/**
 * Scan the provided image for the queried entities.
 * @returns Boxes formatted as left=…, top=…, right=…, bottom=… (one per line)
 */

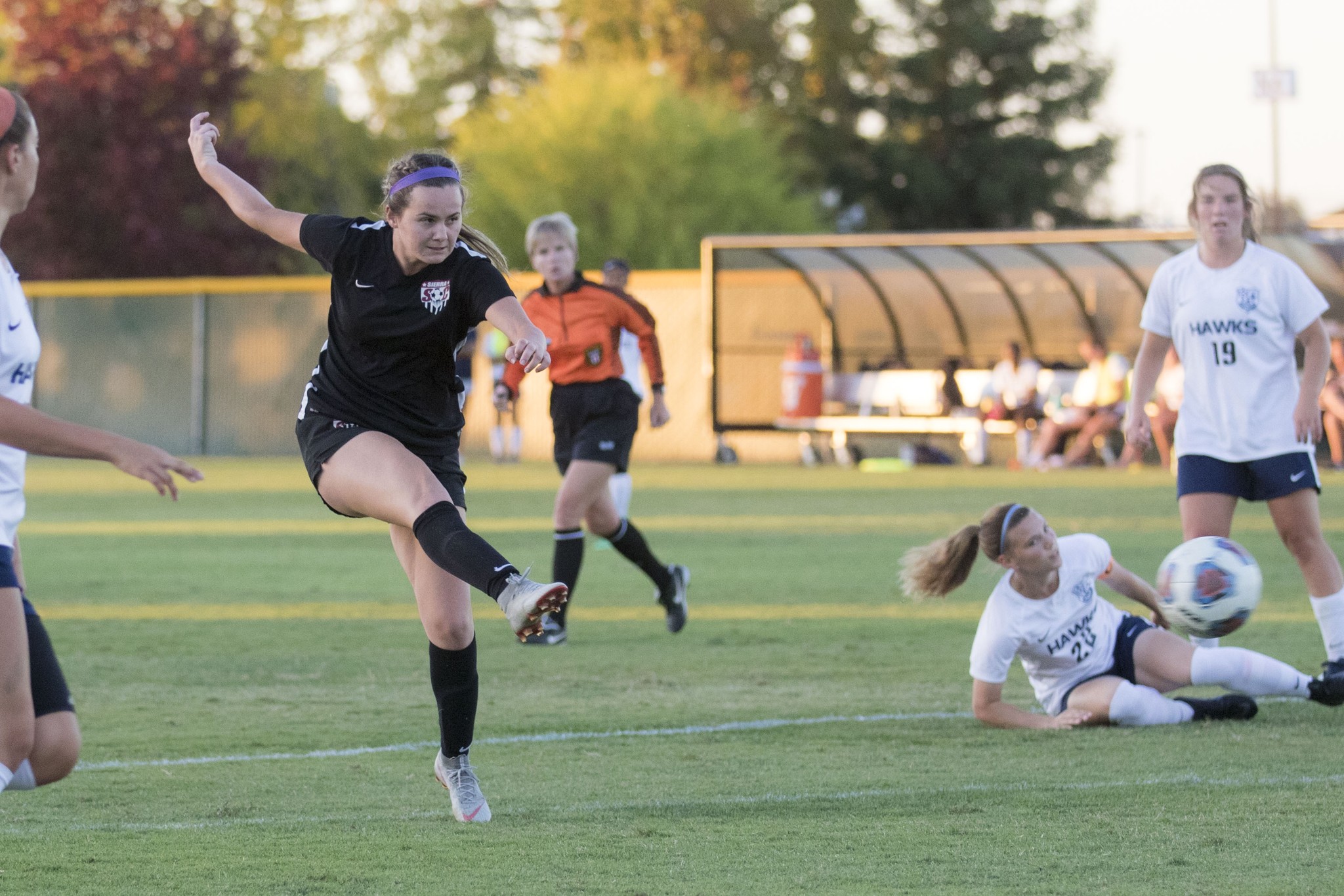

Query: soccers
left=1153, top=534, right=1264, bottom=640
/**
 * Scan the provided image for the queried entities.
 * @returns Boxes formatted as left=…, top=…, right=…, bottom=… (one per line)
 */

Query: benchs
left=768, top=364, right=1074, bottom=471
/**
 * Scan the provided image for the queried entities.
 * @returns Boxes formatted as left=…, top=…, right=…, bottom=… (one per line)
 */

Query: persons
left=483, top=327, right=524, bottom=463
left=1119, top=163, right=1343, bottom=687
left=588, top=256, right=643, bottom=552
left=898, top=500, right=1344, bottom=734
left=493, top=212, right=690, bottom=646
left=766, top=317, right=1344, bottom=468
left=1, top=86, right=205, bottom=804
left=184, top=104, right=569, bottom=826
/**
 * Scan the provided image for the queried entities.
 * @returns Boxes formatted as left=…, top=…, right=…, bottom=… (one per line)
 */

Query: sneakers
left=1306, top=670, right=1344, bottom=706
left=433, top=747, right=492, bottom=824
left=1326, top=658, right=1344, bottom=675
left=657, top=563, right=690, bottom=633
left=1173, top=693, right=1258, bottom=721
left=496, top=573, right=569, bottom=642
left=516, top=615, right=566, bottom=645
